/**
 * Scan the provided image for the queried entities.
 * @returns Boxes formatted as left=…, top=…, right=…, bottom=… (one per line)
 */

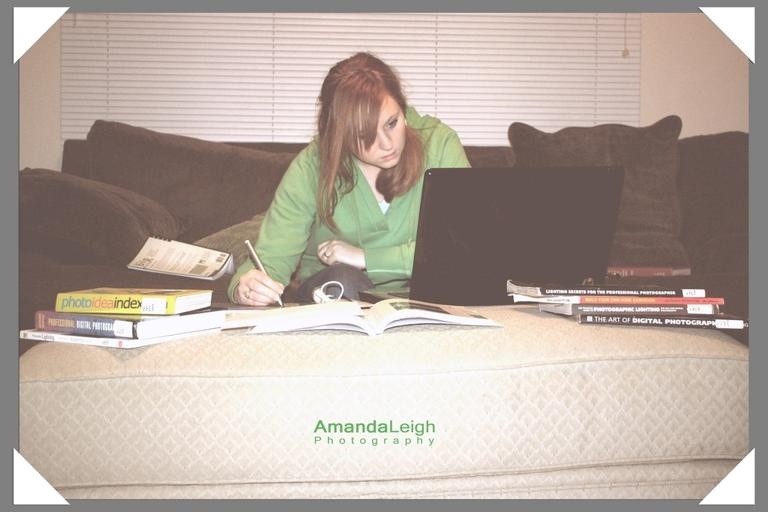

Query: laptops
left=358, top=165, right=624, bottom=306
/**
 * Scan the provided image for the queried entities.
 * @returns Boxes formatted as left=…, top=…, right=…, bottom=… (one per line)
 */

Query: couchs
left=19, top=116, right=748, bottom=357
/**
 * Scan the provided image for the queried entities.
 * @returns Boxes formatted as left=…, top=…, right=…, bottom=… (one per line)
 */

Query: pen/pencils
left=244, top=240, right=283, bottom=307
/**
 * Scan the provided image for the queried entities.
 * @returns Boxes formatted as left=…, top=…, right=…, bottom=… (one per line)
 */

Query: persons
left=229, top=50, right=473, bottom=306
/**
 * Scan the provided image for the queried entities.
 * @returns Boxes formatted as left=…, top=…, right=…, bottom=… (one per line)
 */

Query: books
left=54, top=286, right=214, bottom=316
left=33, top=307, right=227, bottom=340
left=172, top=300, right=282, bottom=331
left=125, top=233, right=233, bottom=282
left=504, top=264, right=745, bottom=334
left=18, top=326, right=222, bottom=349
left=243, top=297, right=504, bottom=337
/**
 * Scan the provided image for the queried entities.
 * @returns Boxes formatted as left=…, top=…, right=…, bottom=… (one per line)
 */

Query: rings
left=323, top=251, right=330, bottom=258
left=246, top=290, right=253, bottom=299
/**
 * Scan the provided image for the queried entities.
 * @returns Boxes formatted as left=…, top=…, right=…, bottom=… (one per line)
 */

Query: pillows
left=19, top=167, right=180, bottom=357
left=86, top=119, right=298, bottom=243
left=508, top=116, right=692, bottom=286
left=191, top=208, right=268, bottom=273
